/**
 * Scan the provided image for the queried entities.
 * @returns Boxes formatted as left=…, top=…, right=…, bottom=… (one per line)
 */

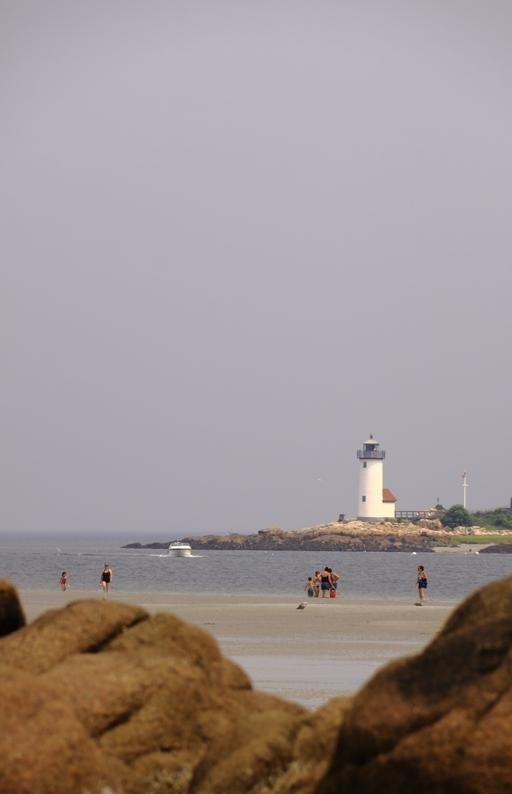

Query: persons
left=317, top=566, right=336, bottom=599
left=58, top=571, right=68, bottom=592
left=327, top=567, right=339, bottom=592
left=414, top=564, right=429, bottom=606
left=304, top=576, right=314, bottom=597
left=99, top=561, right=113, bottom=600
left=312, top=570, right=321, bottom=597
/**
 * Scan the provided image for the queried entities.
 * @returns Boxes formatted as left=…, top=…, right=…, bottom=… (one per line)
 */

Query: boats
left=169, top=541, right=192, bottom=557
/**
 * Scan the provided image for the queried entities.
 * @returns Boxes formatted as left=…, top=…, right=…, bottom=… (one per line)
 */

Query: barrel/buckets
left=330, top=588, right=336, bottom=598
